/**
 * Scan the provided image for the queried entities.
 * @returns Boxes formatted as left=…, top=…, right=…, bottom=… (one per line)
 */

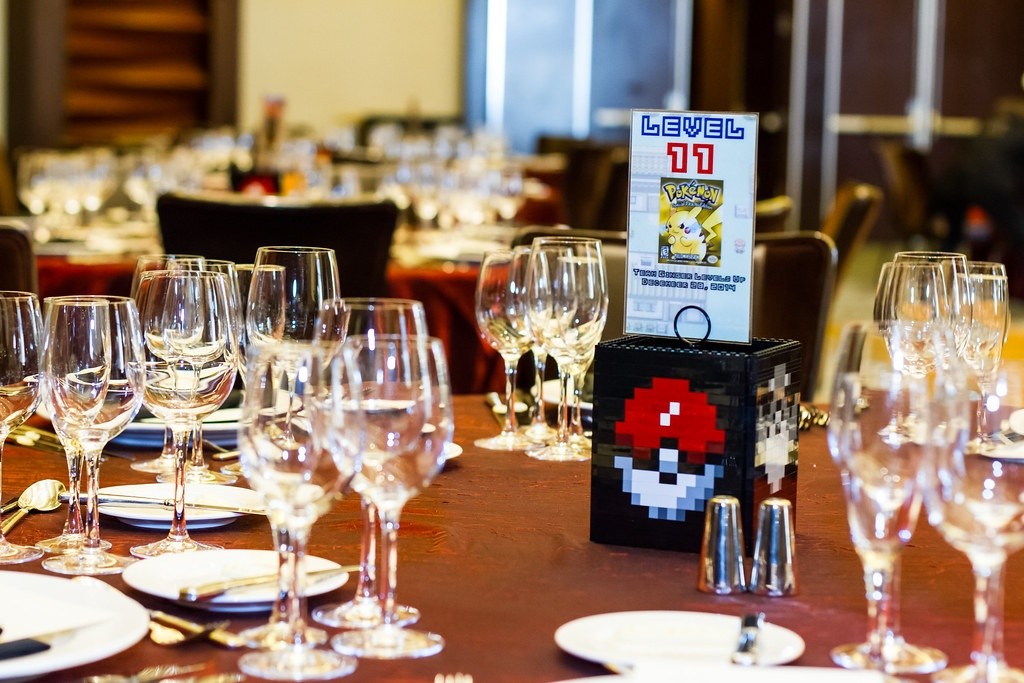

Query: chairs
left=0, top=115, right=1024, bottom=400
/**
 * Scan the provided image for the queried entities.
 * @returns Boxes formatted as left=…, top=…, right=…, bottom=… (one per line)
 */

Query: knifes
left=145, top=607, right=245, bottom=649
left=484, top=392, right=519, bottom=431
left=730, top=610, right=765, bottom=665
left=57, top=490, right=266, bottom=515
left=178, top=563, right=360, bottom=603
left=18, top=424, right=136, bottom=461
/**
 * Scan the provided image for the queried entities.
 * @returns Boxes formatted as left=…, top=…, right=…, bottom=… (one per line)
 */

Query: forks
left=149, top=617, right=232, bottom=649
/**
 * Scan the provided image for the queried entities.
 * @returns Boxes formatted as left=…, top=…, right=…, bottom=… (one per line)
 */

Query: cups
left=17, top=122, right=524, bottom=233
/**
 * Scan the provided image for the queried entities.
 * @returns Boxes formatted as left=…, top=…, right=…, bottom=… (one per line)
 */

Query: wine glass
left=0, top=248, right=456, bottom=681
left=912, top=331, right=1024, bottom=683
left=875, top=249, right=1011, bottom=453
left=474, top=235, right=610, bottom=462
left=825, top=318, right=971, bottom=675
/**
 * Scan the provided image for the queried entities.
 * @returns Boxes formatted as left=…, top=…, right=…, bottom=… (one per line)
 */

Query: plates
left=122, top=547, right=350, bottom=612
left=110, top=388, right=303, bottom=449
left=96, top=483, right=256, bottom=530
left=553, top=607, right=806, bottom=665
left=0, top=570, right=149, bottom=679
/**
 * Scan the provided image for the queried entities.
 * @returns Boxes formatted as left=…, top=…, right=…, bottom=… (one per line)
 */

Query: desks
left=0, top=392, right=1024, bottom=683
left=39, top=253, right=522, bottom=394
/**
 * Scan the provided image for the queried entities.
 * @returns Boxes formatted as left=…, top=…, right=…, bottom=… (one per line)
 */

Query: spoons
left=0, top=479, right=66, bottom=534
